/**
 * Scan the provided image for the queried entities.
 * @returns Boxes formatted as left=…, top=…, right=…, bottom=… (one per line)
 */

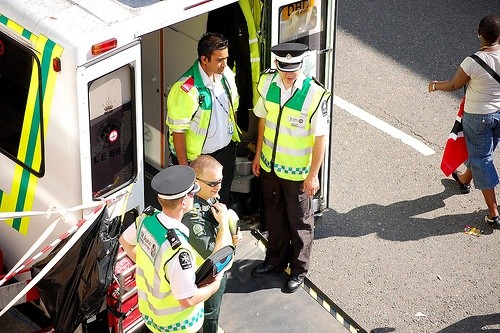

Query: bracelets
left=433, top=84, right=435, bottom=89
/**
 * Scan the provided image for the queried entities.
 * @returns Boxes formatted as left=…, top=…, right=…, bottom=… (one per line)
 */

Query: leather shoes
left=252, top=261, right=288, bottom=276
left=285, top=272, right=303, bottom=292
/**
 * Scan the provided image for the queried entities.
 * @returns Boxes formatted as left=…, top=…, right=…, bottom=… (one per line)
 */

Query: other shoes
left=452, top=169, right=470, bottom=193
left=484, top=214, right=500, bottom=229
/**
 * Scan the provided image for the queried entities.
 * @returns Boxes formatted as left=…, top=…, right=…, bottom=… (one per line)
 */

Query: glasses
left=197, top=177, right=223, bottom=187
left=216, top=40, right=229, bottom=46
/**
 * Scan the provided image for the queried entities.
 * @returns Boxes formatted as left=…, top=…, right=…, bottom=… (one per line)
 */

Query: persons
left=165, top=33, right=242, bottom=205
left=119, top=165, right=224, bottom=333
left=428, top=15, right=500, bottom=232
left=252, top=43, right=332, bottom=292
left=181, top=156, right=243, bottom=333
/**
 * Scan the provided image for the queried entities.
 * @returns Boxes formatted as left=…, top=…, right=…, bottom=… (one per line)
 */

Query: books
left=228, top=208, right=240, bottom=235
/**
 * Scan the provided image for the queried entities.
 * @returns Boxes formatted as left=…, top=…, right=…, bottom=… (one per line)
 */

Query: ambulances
left=1, top=0, right=338, bottom=333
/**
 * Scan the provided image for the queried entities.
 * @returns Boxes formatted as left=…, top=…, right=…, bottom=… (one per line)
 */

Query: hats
left=270, top=43, right=309, bottom=72
left=151, top=165, right=201, bottom=200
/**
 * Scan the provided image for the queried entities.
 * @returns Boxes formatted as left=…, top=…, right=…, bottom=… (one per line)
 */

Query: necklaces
left=479, top=45, right=497, bottom=49
